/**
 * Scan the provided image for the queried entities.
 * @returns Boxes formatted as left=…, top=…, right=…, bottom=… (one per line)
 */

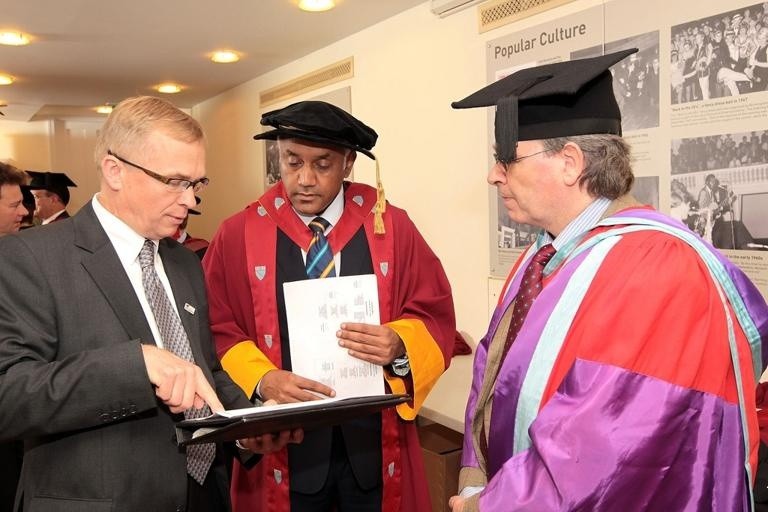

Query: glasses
left=493, top=142, right=566, bottom=173
left=107, top=149, right=210, bottom=197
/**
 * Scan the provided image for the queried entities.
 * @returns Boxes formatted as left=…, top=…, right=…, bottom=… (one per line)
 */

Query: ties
left=303, top=216, right=340, bottom=281
left=137, top=239, right=217, bottom=489
left=477, top=242, right=558, bottom=467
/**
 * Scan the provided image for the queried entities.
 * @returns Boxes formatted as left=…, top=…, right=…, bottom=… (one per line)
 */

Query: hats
left=251, top=101, right=379, bottom=162
left=23, top=170, right=78, bottom=206
left=450, top=47, right=639, bottom=164
left=187, top=197, right=203, bottom=216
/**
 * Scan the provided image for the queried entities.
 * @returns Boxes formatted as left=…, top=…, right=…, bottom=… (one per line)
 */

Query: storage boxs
left=420, top=423, right=464, bottom=512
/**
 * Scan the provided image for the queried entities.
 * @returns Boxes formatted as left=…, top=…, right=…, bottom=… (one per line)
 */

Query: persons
left=0, top=96, right=302, bottom=512
left=24, top=166, right=77, bottom=224
left=449, top=47, right=767, bottom=512
left=202, top=101, right=455, bottom=512
left=667, top=174, right=754, bottom=249
left=19, top=186, right=36, bottom=228
left=671, top=3, right=768, bottom=104
left=0, top=161, right=28, bottom=235
left=610, top=43, right=658, bottom=120
left=172, top=196, right=211, bottom=256
